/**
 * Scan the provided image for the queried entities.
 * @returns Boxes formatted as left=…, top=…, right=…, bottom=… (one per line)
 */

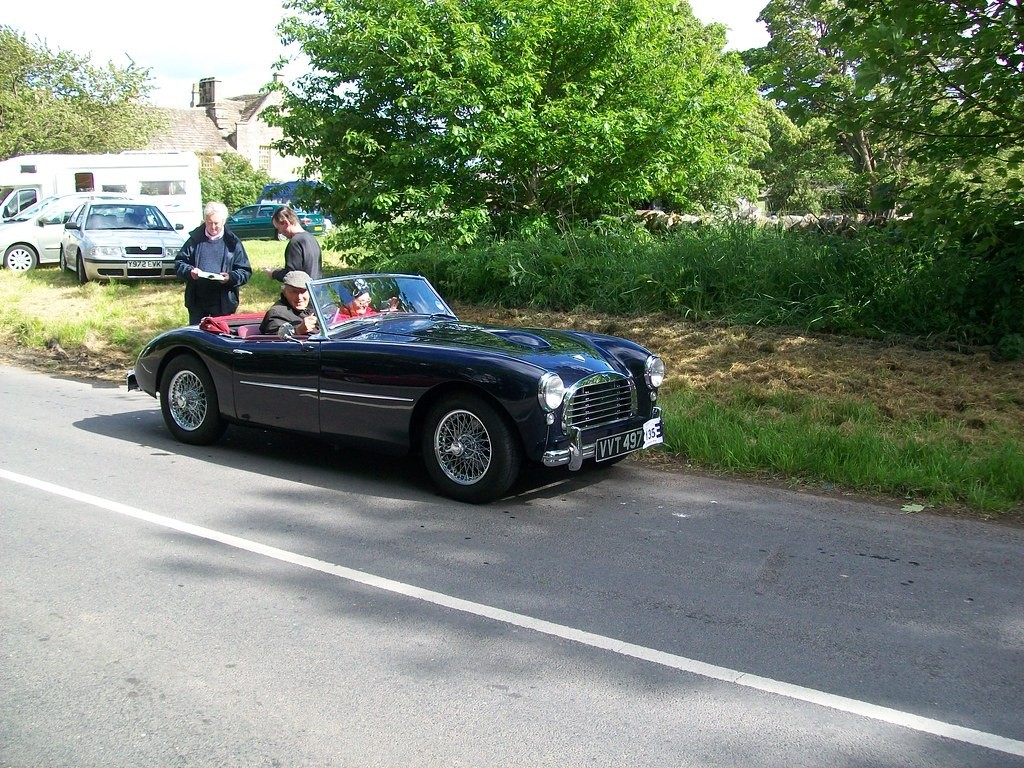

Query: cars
left=225, top=203, right=327, bottom=243
left=0, top=190, right=173, bottom=273
left=59, top=199, right=188, bottom=287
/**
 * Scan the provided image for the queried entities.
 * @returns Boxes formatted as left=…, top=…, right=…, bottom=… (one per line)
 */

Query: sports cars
left=127, top=272, right=665, bottom=505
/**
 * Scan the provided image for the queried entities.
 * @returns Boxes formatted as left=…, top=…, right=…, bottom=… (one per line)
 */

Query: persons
left=261, top=206, right=322, bottom=284
left=173, top=201, right=253, bottom=325
left=259, top=269, right=401, bottom=335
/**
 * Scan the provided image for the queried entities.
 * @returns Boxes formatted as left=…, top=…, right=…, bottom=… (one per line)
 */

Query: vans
left=0, top=149, right=203, bottom=244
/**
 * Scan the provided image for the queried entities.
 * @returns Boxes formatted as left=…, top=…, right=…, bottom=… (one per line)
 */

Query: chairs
left=91, top=213, right=140, bottom=226
